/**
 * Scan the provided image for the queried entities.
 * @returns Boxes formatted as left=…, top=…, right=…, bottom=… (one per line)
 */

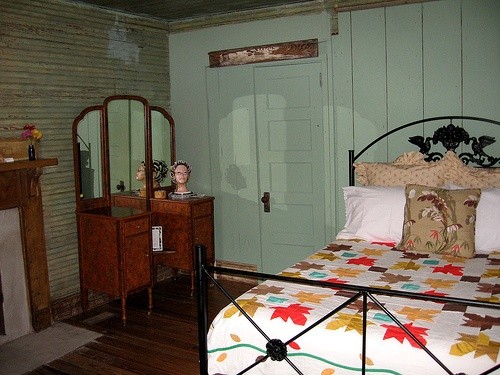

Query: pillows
left=336, top=151, right=500, bottom=258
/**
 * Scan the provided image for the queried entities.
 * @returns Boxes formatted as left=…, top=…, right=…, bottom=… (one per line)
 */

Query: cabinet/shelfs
left=73, top=191, right=215, bottom=325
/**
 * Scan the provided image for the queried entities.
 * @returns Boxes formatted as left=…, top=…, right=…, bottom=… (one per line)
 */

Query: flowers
left=21, top=125, right=43, bottom=145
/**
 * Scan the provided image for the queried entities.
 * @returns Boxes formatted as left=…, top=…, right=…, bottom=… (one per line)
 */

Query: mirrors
left=74, top=95, right=175, bottom=200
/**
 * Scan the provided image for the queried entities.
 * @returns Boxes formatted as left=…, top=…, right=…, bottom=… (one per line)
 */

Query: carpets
left=0, top=321, right=104, bottom=375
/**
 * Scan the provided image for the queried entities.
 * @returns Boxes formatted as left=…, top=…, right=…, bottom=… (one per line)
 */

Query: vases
left=28, top=145, right=36, bottom=161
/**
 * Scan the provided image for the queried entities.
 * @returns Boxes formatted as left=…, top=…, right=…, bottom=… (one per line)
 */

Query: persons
left=169, top=160, right=197, bottom=198
left=136, top=160, right=168, bottom=198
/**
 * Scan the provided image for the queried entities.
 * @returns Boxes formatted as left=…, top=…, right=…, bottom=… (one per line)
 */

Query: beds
left=194, top=115, right=500, bottom=375
